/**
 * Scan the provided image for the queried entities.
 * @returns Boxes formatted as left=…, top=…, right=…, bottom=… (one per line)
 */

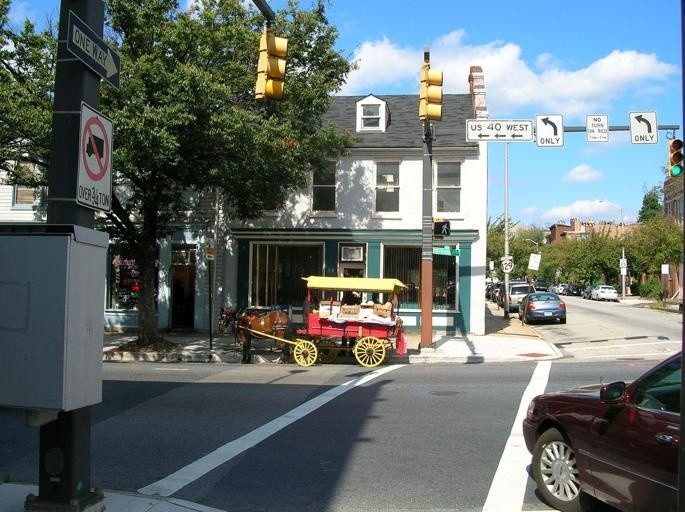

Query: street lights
left=522, top=238, right=538, bottom=252
left=599, top=200, right=626, bottom=296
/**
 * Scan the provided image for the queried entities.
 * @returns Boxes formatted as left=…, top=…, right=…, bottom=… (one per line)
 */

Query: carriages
left=218, top=276, right=409, bottom=367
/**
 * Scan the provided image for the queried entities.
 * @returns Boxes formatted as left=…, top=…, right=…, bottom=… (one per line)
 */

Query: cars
left=583, top=285, right=618, bottom=302
left=486, top=280, right=566, bottom=325
left=523, top=350, right=685, bottom=512
left=555, top=284, right=581, bottom=296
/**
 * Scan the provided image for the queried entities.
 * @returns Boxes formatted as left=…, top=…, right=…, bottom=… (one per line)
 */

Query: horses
left=218, top=306, right=291, bottom=364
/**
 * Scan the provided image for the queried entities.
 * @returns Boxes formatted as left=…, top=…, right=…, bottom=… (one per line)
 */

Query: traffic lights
left=255, top=36, right=289, bottom=99
left=667, top=137, right=683, bottom=178
left=418, top=69, right=444, bottom=122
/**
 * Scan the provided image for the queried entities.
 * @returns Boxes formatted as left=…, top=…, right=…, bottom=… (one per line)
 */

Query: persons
left=366, top=292, right=380, bottom=304
left=339, top=291, right=361, bottom=357
